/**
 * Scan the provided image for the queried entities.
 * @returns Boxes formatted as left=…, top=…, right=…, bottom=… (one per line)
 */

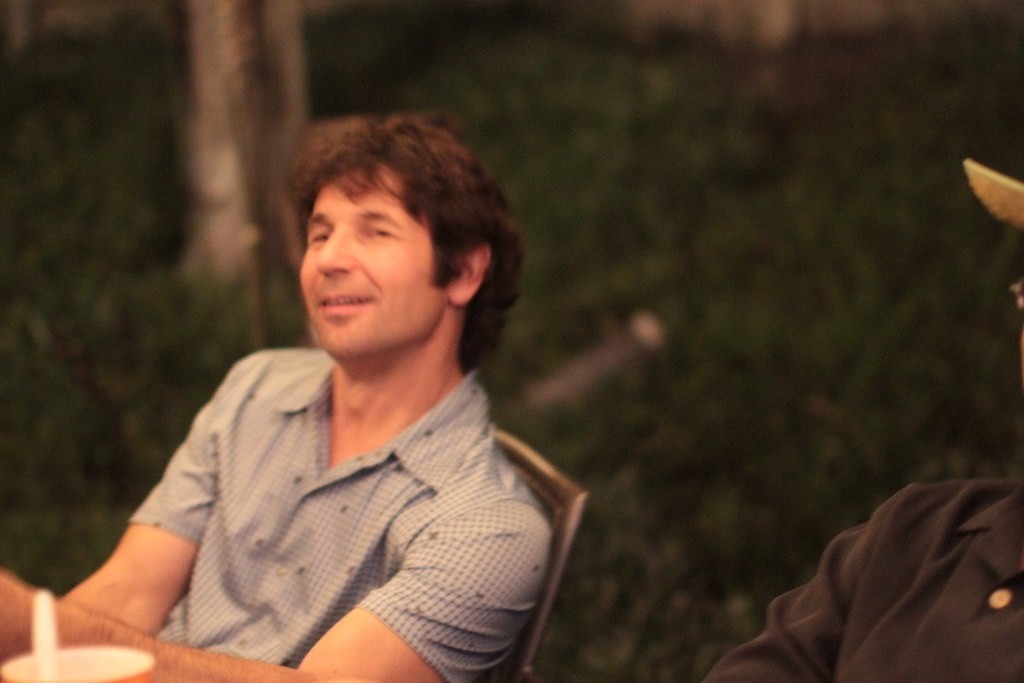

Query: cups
left=0, top=643, right=157, bottom=683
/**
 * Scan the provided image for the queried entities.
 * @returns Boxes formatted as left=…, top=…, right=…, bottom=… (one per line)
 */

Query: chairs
left=496, top=426, right=592, bottom=683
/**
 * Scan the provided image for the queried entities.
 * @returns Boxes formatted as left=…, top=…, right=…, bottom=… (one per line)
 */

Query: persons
left=699, top=157, right=1024, bottom=683
left=0, top=112, right=551, bottom=683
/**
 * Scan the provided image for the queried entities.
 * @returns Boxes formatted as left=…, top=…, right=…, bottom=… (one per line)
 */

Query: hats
left=962, top=159, right=1024, bottom=231
